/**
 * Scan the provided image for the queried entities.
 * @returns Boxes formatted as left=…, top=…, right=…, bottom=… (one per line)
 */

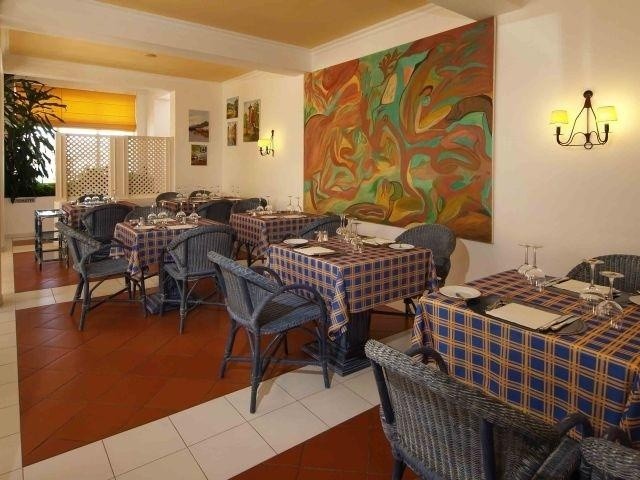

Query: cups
left=68, top=200, right=78, bottom=206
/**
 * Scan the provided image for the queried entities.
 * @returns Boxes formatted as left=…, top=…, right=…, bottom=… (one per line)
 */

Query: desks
left=419, top=267, right=639, bottom=447
left=112, top=215, right=227, bottom=316
left=268, top=230, right=434, bottom=377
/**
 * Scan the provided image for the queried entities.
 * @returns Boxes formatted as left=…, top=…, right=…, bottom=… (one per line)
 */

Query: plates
left=437, top=283, right=482, bottom=302
left=360, top=236, right=416, bottom=251
left=281, top=237, right=309, bottom=247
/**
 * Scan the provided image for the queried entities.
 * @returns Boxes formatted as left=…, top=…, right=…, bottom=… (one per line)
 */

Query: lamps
left=550, top=90, right=617, bottom=150
left=256, top=130, right=274, bottom=159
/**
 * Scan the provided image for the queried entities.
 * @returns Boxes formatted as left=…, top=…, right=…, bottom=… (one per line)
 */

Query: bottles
left=315, top=231, right=328, bottom=243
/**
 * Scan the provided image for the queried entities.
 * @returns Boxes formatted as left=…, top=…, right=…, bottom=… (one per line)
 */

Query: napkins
left=486, top=303, right=561, bottom=330
left=554, top=279, right=618, bottom=299
left=362, top=236, right=394, bottom=247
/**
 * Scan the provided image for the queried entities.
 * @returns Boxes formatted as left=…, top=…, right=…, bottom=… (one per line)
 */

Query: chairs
left=366, top=224, right=455, bottom=342
left=208, top=251, right=331, bottom=413
left=55, top=221, right=147, bottom=331
left=364, top=338, right=594, bottom=479
left=578, top=428, right=639, bottom=478
left=564, top=254, right=639, bottom=295
left=56, top=193, right=348, bottom=288
left=159, top=225, right=238, bottom=333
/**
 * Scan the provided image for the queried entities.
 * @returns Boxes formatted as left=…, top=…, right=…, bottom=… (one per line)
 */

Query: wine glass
left=173, top=183, right=243, bottom=202
left=574, top=256, right=625, bottom=335
left=123, top=199, right=199, bottom=231
left=335, top=212, right=365, bottom=256
left=84, top=188, right=119, bottom=208
left=517, top=241, right=548, bottom=294
left=248, top=194, right=307, bottom=220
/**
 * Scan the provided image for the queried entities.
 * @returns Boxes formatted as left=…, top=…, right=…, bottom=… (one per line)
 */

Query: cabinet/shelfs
left=35, top=208, right=69, bottom=271
